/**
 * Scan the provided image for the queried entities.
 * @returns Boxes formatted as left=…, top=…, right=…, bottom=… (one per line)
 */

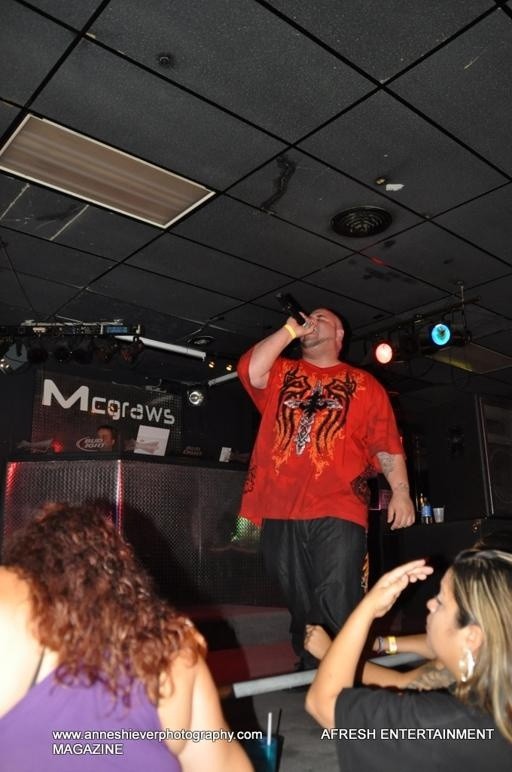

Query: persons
left=96, top=425, right=118, bottom=451
left=238, top=307, right=416, bottom=693
left=299, top=312, right=311, bottom=324
left=304, top=547, right=512, bottom=772
left=303, top=511, right=512, bottom=692
left=0, top=501, right=256, bottom=772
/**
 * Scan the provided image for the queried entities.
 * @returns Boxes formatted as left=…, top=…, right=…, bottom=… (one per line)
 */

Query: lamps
left=1, top=331, right=143, bottom=365
left=430, top=322, right=473, bottom=348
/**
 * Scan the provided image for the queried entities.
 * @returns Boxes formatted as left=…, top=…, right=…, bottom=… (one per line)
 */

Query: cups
left=254, top=736, right=277, bottom=772
left=433, top=507, right=445, bottom=523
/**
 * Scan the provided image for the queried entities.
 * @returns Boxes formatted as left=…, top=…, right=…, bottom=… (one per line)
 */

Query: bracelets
left=282, top=324, right=296, bottom=339
left=376, top=636, right=384, bottom=654
left=385, top=636, right=397, bottom=655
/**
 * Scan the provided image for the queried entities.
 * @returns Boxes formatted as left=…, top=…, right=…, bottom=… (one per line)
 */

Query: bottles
left=420, top=493, right=433, bottom=524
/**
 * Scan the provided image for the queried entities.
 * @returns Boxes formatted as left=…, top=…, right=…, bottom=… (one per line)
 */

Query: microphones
left=284, top=304, right=311, bottom=330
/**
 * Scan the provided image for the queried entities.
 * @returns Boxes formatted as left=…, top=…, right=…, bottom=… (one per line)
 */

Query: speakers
left=0, top=365, right=286, bottom=608
left=380, top=385, right=512, bottom=634
